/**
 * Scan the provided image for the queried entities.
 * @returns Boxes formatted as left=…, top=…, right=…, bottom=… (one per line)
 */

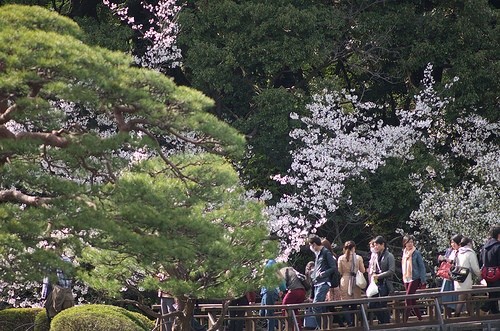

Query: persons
left=454, top=237, right=480, bottom=317
left=338, top=241, right=365, bottom=327
left=439, top=234, right=463, bottom=318
left=367, top=236, right=395, bottom=323
left=303, top=235, right=341, bottom=329
left=157, top=273, right=176, bottom=313
left=228, top=291, right=256, bottom=331
left=260, top=260, right=279, bottom=331
left=402, top=236, right=426, bottom=321
left=275, top=263, right=306, bottom=331
left=41, top=248, right=75, bottom=317
left=481, top=227, right=500, bottom=315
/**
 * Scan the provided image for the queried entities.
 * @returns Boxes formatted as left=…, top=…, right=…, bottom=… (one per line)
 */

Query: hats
left=372, top=235, right=384, bottom=244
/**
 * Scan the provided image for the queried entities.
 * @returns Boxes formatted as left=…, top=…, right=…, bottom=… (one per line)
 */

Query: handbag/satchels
left=479, top=266, right=500, bottom=283
left=436, top=261, right=454, bottom=281
left=326, top=268, right=340, bottom=288
left=448, top=265, right=470, bottom=283
left=355, top=270, right=367, bottom=290
left=293, top=269, right=311, bottom=290
